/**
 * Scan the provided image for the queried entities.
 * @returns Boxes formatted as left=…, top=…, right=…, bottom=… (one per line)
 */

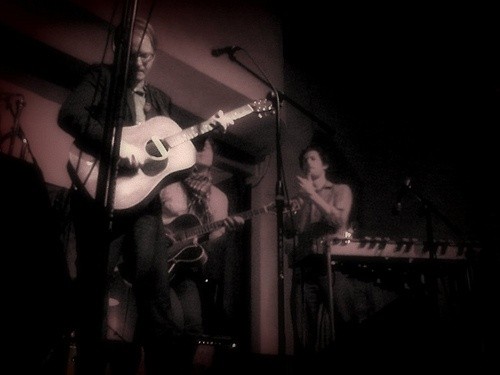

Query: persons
left=155, top=137, right=245, bottom=338
left=281, top=147, right=358, bottom=361
left=55, top=18, right=235, bottom=340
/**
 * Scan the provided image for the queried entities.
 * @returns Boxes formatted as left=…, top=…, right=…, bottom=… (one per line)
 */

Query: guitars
left=107, top=199, right=302, bottom=293
left=67, top=99, right=280, bottom=212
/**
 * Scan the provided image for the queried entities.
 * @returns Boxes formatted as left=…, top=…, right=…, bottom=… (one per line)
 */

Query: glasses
left=132, top=50, right=154, bottom=62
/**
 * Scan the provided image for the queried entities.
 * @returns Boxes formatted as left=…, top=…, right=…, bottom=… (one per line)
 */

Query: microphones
left=212, top=44, right=242, bottom=57
left=14, top=99, right=27, bottom=134
